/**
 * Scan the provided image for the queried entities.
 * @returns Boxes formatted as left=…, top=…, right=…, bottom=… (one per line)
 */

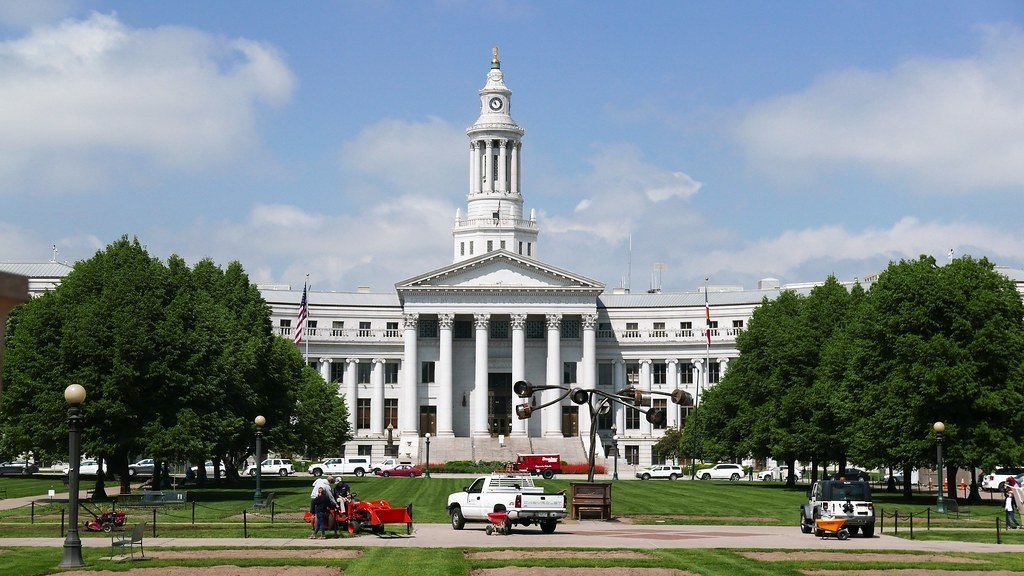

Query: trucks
left=503, top=453, right=561, bottom=480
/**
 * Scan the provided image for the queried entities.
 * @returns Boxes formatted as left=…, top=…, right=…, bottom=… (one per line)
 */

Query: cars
left=190, top=460, right=237, bottom=477
left=827, top=468, right=870, bottom=483
left=883, top=469, right=904, bottom=484
left=377, top=464, right=423, bottom=479
left=0, top=460, right=39, bottom=474
left=63, top=459, right=107, bottom=476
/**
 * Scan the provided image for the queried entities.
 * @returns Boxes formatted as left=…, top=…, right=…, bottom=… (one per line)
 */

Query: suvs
left=128, top=458, right=167, bottom=476
left=242, top=458, right=296, bottom=477
left=696, top=463, right=745, bottom=482
left=757, top=465, right=802, bottom=484
left=636, top=464, right=684, bottom=482
left=977, top=464, right=1024, bottom=502
left=799, top=479, right=876, bottom=538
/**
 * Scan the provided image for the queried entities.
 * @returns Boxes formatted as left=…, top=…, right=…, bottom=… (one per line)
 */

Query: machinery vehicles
left=78, top=499, right=128, bottom=533
left=303, top=492, right=414, bottom=536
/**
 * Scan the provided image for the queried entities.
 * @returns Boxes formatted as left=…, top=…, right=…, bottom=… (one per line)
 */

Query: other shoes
left=1016, top=525, right=1021, bottom=530
left=308, top=534, right=316, bottom=539
left=319, top=534, right=326, bottom=539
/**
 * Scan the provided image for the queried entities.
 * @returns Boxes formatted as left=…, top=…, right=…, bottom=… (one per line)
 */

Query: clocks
left=489, top=97, right=502, bottom=111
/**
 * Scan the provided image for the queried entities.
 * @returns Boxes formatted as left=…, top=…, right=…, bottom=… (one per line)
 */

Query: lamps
left=47, top=490, right=55, bottom=496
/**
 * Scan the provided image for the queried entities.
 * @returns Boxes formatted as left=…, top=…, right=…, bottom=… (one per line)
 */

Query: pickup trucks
left=371, top=458, right=416, bottom=476
left=308, top=455, right=373, bottom=478
left=446, top=474, right=568, bottom=534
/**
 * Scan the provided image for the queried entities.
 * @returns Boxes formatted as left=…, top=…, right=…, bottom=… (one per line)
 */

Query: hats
left=334, top=477, right=342, bottom=485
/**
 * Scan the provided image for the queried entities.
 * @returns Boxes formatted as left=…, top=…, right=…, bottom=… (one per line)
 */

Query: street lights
left=514, top=379, right=692, bottom=484
left=253, top=415, right=266, bottom=510
left=424, top=433, right=431, bottom=478
left=933, top=421, right=945, bottom=514
left=57, top=382, right=87, bottom=570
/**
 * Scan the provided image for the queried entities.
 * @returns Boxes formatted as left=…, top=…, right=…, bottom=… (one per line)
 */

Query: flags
left=294, top=278, right=307, bottom=347
left=706, top=281, right=711, bottom=346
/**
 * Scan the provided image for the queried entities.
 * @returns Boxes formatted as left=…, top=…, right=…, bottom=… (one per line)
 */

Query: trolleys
left=801, top=518, right=849, bottom=541
left=485, top=510, right=520, bottom=535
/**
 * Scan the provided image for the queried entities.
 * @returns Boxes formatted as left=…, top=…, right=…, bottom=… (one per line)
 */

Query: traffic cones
left=944, top=477, right=948, bottom=489
left=928, top=477, right=934, bottom=488
left=959, top=478, right=966, bottom=490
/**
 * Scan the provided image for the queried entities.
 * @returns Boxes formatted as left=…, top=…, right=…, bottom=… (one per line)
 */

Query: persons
left=182, top=467, right=195, bottom=483
left=334, top=477, right=350, bottom=501
left=308, top=487, right=339, bottom=539
left=1003, top=477, right=1020, bottom=529
left=748, top=465, right=753, bottom=481
left=310, top=476, right=340, bottom=513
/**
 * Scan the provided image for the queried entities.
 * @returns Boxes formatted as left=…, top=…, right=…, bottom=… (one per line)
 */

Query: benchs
left=61, top=471, right=69, bottom=487
left=86, top=488, right=95, bottom=499
left=942, top=499, right=971, bottom=521
left=254, top=492, right=274, bottom=517
left=109, top=522, right=146, bottom=564
left=0, top=487, right=7, bottom=499
left=579, top=507, right=604, bottom=520
left=118, top=490, right=187, bottom=514
